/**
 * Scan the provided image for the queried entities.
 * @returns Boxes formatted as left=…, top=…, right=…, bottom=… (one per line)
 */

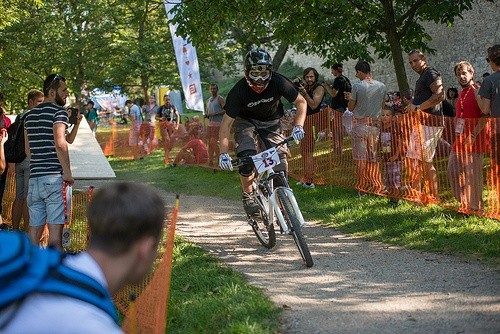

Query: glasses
left=355, top=73, right=358, bottom=78
left=48, top=74, right=61, bottom=88
left=486, top=57, right=490, bottom=62
left=249, top=69, right=270, bottom=81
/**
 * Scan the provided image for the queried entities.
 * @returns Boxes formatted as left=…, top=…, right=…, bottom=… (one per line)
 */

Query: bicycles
left=217, top=129, right=315, bottom=268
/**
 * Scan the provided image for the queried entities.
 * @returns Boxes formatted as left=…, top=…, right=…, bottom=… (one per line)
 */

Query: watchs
left=415, top=105, right=418, bottom=111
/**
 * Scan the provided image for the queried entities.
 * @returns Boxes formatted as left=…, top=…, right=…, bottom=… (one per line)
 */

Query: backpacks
left=4, top=112, right=31, bottom=163
left=0, top=231, right=120, bottom=328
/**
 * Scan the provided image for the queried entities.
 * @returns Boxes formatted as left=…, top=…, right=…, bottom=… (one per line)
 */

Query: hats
left=332, top=64, right=342, bottom=72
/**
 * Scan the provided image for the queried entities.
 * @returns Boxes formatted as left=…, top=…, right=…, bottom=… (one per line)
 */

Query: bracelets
left=470, top=132, right=475, bottom=138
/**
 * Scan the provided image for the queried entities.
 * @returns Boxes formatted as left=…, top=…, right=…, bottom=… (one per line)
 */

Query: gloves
left=291, top=125, right=304, bottom=144
left=219, top=153, right=233, bottom=172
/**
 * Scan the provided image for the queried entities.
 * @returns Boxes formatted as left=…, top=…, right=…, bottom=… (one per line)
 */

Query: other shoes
left=170, top=162, right=176, bottom=167
left=165, top=164, right=170, bottom=168
left=442, top=198, right=462, bottom=208
left=303, top=182, right=315, bottom=188
left=356, top=190, right=367, bottom=196
left=389, top=199, right=402, bottom=207
left=457, top=207, right=478, bottom=217
left=296, top=178, right=305, bottom=186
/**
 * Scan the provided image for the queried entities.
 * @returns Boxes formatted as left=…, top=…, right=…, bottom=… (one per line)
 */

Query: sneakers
left=276, top=211, right=287, bottom=226
left=242, top=191, right=259, bottom=214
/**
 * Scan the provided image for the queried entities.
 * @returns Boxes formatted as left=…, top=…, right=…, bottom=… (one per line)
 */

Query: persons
left=171, top=127, right=208, bottom=168
left=206, top=84, right=226, bottom=168
left=219, top=48, right=308, bottom=231
left=344, top=60, right=387, bottom=198
left=0, top=91, right=11, bottom=229
left=321, top=63, right=352, bottom=165
left=472, top=44, right=500, bottom=199
left=442, top=88, right=458, bottom=117
left=385, top=89, right=414, bottom=113
left=0, top=181, right=165, bottom=334
left=23, top=73, right=74, bottom=248
left=6, top=89, right=44, bottom=232
left=65, top=101, right=99, bottom=145
left=447, top=61, right=490, bottom=213
left=403, top=49, right=445, bottom=201
left=155, top=94, right=180, bottom=164
left=291, top=67, right=325, bottom=189
left=179, top=115, right=203, bottom=147
left=125, top=100, right=143, bottom=151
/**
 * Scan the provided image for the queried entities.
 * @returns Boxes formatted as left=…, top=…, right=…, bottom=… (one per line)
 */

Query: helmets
left=244, top=48, right=272, bottom=94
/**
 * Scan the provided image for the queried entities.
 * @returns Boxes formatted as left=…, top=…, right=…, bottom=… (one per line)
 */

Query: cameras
left=294, top=77, right=304, bottom=87
